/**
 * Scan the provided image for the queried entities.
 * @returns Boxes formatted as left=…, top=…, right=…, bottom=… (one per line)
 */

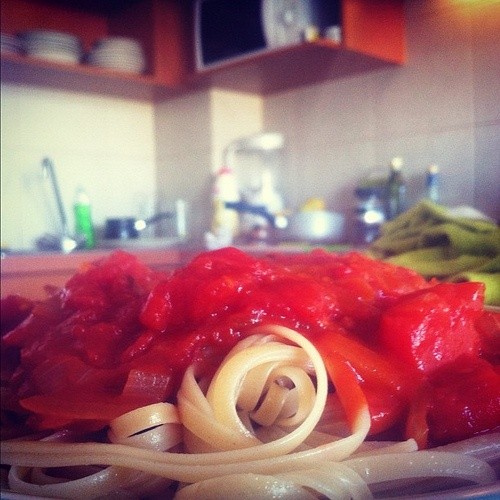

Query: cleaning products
left=71, top=190, right=96, bottom=250
left=352, top=155, right=408, bottom=247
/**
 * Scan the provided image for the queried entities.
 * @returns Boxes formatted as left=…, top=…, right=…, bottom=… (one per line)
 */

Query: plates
left=0, top=24, right=146, bottom=78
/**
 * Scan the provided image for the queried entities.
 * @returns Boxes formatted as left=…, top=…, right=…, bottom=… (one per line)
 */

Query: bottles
left=74, top=186, right=96, bottom=249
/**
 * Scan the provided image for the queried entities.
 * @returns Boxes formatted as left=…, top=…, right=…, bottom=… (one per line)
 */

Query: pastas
left=0, top=246, right=499, bottom=500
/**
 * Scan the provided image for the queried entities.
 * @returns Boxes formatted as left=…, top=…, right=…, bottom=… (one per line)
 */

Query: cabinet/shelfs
left=2, top=1, right=192, bottom=103
left=192, top=1, right=408, bottom=98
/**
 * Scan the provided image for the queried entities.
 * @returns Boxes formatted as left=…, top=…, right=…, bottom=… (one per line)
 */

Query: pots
left=222, top=200, right=346, bottom=244
left=105, top=208, right=176, bottom=240
